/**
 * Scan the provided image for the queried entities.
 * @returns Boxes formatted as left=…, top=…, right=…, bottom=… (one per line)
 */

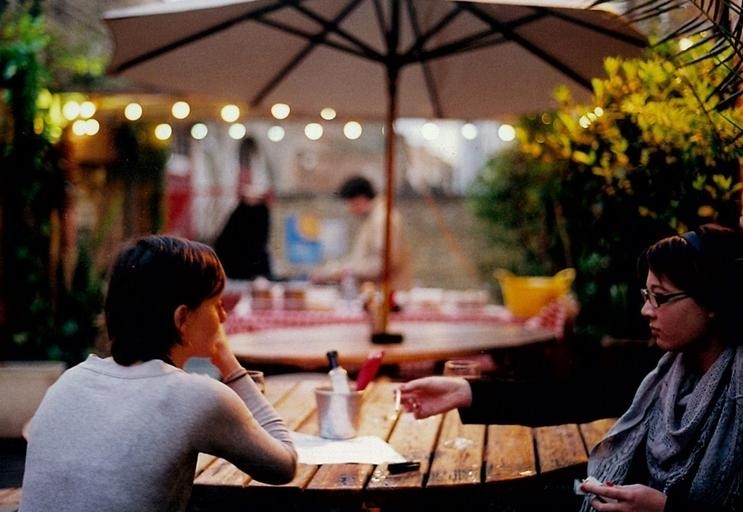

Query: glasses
left=640, top=287, right=691, bottom=309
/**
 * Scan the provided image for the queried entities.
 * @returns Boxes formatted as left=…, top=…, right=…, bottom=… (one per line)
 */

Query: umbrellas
left=100, top=1, right=647, bottom=337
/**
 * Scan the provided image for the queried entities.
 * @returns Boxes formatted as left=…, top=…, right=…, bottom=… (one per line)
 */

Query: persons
left=308, top=173, right=407, bottom=294
left=213, top=137, right=289, bottom=282
left=18, top=234, right=296, bottom=511
left=392, top=223, right=742, bottom=511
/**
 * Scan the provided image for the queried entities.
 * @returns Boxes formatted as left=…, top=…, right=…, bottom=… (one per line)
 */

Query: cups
left=314, top=384, right=363, bottom=439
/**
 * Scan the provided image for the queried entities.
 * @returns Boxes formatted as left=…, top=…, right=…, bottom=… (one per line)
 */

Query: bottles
left=326, top=351, right=350, bottom=392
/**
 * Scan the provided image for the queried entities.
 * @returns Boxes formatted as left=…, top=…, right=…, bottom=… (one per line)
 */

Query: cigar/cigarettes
left=394, top=385, right=401, bottom=412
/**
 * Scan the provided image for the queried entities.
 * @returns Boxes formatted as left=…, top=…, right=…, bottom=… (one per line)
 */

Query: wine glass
left=443, top=360, right=477, bottom=449
